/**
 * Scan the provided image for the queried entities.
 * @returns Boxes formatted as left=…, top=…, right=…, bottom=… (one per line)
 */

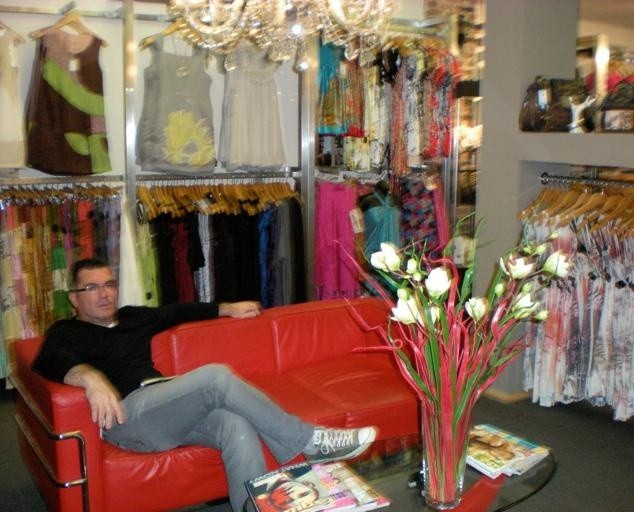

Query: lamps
left=153, top=0, right=401, bottom=80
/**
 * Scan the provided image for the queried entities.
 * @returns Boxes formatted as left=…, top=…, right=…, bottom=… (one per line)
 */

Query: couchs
left=3, top=288, right=430, bottom=512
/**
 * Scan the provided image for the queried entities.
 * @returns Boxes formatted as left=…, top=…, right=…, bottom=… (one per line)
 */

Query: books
left=244, top=456, right=390, bottom=512
left=443, top=421, right=553, bottom=479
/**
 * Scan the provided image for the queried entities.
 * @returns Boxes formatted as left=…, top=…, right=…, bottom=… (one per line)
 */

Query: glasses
left=66, top=279, right=118, bottom=294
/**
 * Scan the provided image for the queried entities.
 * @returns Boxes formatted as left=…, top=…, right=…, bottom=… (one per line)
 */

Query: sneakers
left=303, top=423, right=378, bottom=467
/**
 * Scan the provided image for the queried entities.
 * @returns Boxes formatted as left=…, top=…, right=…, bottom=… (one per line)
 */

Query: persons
left=30, top=256, right=379, bottom=511
left=261, top=469, right=321, bottom=510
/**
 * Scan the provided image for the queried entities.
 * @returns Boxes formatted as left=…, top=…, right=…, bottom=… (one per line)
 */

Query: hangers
left=137, top=17, right=195, bottom=49
left=29, top=6, right=107, bottom=49
left=370, top=31, right=452, bottom=54
left=313, top=160, right=442, bottom=189
left=0, top=19, right=27, bottom=44
left=512, top=172, right=634, bottom=246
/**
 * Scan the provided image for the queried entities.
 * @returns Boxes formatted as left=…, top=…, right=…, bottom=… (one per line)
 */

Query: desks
left=241, top=429, right=562, bottom=510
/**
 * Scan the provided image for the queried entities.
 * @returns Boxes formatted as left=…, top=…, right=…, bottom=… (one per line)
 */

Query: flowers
left=328, top=211, right=576, bottom=500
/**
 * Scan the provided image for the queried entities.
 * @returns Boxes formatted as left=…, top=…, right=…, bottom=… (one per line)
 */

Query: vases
left=411, top=399, right=473, bottom=507
